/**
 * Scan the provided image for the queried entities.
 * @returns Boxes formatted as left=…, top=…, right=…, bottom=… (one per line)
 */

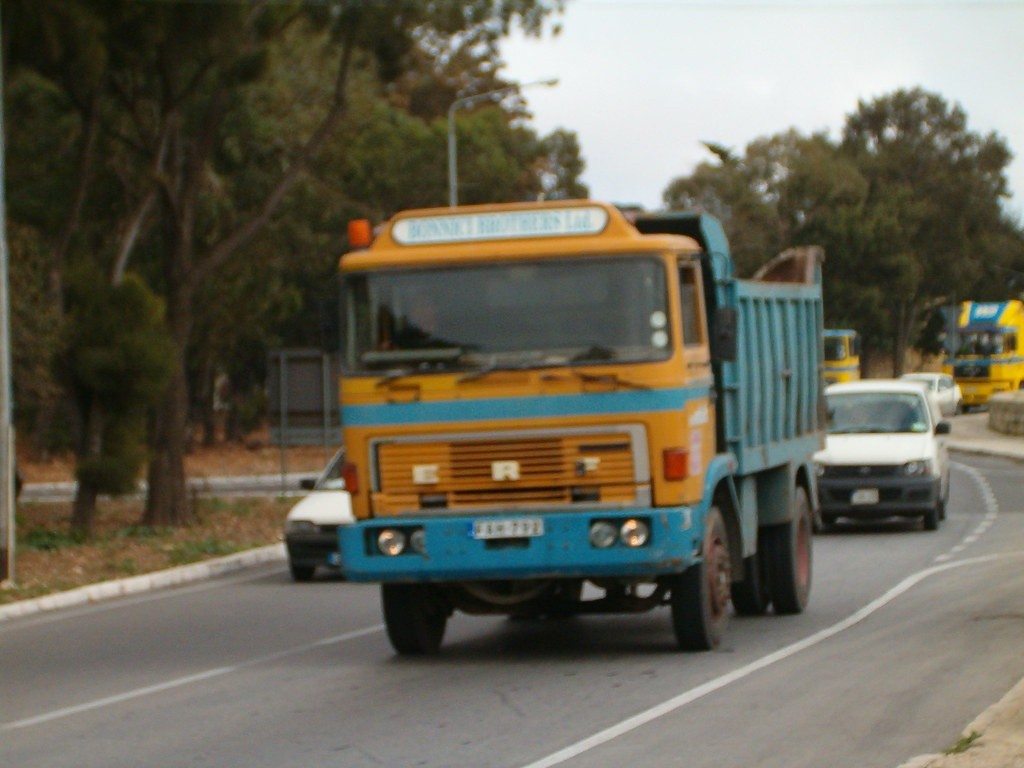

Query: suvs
left=811, top=380, right=953, bottom=532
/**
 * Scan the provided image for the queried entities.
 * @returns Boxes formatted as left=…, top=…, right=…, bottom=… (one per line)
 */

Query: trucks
left=939, top=297, right=1023, bottom=413
left=315, top=198, right=828, bottom=657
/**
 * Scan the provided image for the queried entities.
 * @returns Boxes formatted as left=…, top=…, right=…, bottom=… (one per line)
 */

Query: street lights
left=448, top=76, right=561, bottom=208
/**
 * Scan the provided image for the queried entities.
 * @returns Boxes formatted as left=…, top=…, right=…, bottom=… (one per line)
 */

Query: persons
left=380, top=290, right=439, bottom=352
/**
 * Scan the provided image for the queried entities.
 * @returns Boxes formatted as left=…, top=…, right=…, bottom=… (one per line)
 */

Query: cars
left=283, top=444, right=358, bottom=580
left=896, top=372, right=963, bottom=415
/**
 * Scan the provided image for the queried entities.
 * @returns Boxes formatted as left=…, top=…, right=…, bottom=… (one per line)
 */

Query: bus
left=820, top=328, right=862, bottom=387
